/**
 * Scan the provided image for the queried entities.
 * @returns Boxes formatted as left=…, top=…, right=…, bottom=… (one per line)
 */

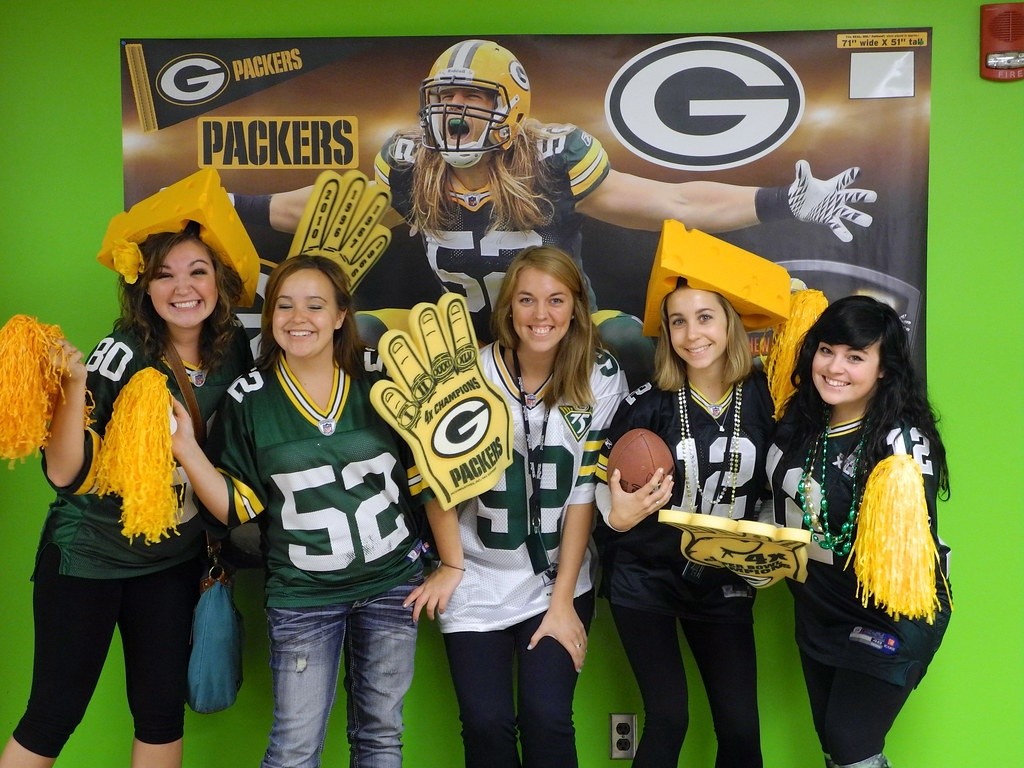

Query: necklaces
left=677, top=368, right=743, bottom=523
left=797, top=407, right=872, bottom=561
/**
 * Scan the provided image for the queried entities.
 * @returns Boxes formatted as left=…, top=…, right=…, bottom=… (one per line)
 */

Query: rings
left=656, top=500, right=661, bottom=508
left=575, top=644, right=581, bottom=648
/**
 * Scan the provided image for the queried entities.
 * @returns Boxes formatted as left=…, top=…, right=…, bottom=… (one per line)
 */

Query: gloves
left=777, top=160, right=878, bottom=243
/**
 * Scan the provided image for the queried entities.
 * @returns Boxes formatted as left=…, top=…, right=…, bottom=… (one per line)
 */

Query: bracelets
left=440, top=561, right=466, bottom=572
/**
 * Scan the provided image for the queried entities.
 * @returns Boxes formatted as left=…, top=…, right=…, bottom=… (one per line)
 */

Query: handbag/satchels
left=187, top=559, right=244, bottom=716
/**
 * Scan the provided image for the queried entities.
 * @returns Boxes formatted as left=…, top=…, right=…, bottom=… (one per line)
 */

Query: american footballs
left=606, top=428, right=674, bottom=498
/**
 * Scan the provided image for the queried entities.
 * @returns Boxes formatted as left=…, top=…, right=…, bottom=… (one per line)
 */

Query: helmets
left=418, top=39, right=531, bottom=167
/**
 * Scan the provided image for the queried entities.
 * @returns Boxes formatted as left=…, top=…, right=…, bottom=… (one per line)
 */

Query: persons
left=161, top=36, right=879, bottom=404
left=429, top=244, right=631, bottom=768
left=765, top=293, right=954, bottom=768
left=158, top=251, right=465, bottom=768
left=0, top=218, right=257, bottom=768
left=593, top=273, right=778, bottom=768
